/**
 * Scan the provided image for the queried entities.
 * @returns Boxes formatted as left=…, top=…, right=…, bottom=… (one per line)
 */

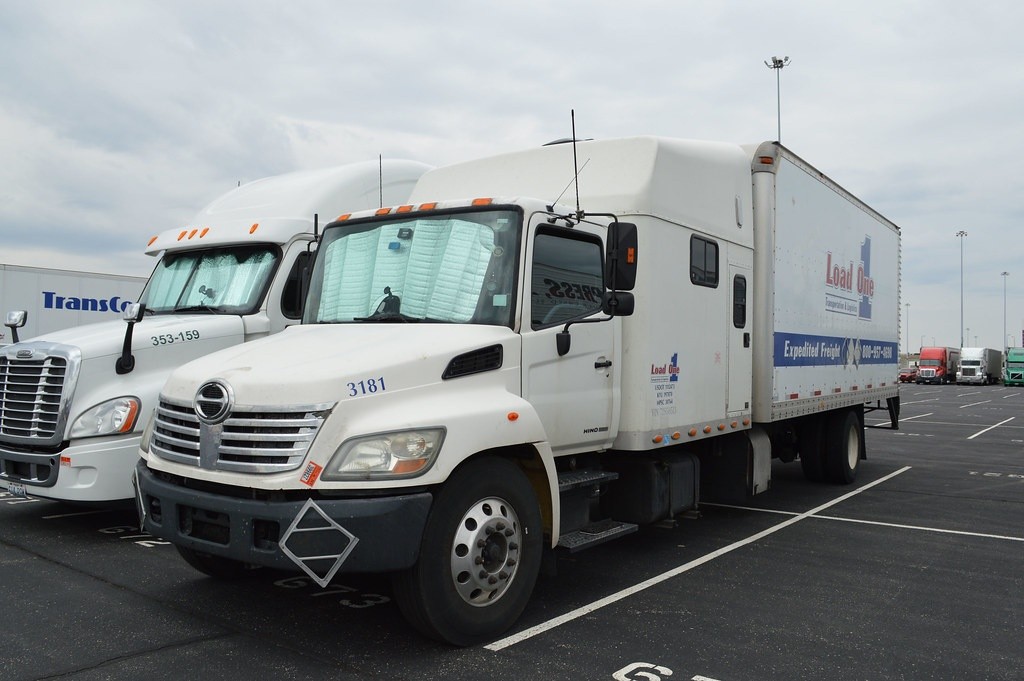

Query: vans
left=899, top=367, right=916, bottom=383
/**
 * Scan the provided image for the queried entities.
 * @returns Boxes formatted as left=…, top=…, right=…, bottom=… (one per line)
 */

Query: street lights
left=904, top=302, right=910, bottom=354
left=956, top=229, right=968, bottom=349
left=1001, top=271, right=1010, bottom=351
left=763, top=54, right=792, bottom=145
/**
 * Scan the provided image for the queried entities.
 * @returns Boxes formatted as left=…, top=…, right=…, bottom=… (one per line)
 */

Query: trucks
left=955, top=346, right=1001, bottom=385
left=915, top=346, right=960, bottom=385
left=1002, top=346, right=1024, bottom=387
left=0, top=136, right=902, bottom=649
